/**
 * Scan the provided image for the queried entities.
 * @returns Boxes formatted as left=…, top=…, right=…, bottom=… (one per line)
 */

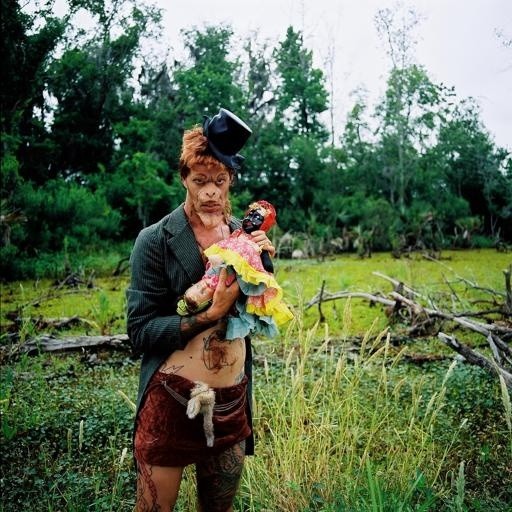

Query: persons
left=125, top=121, right=276, bottom=512
left=174, top=200, right=294, bottom=339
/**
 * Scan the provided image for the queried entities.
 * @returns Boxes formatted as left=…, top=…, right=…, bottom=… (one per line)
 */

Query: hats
left=202, top=108, right=253, bottom=169
